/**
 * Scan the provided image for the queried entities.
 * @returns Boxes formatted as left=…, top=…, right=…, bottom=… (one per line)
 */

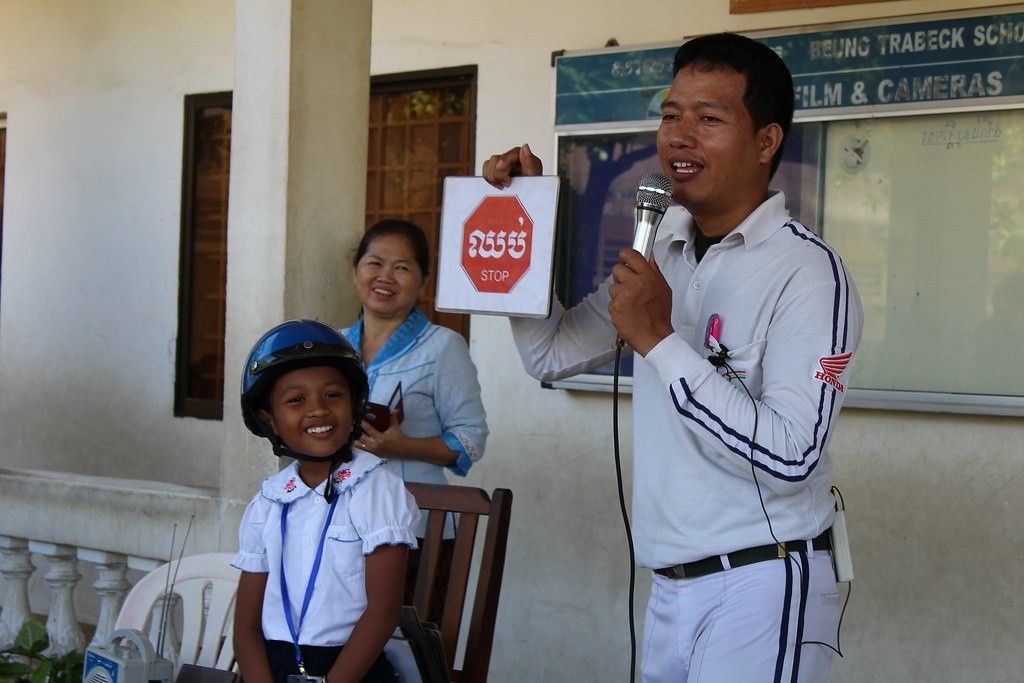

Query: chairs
left=405, top=482, right=513, bottom=683
left=115, top=551, right=242, bottom=683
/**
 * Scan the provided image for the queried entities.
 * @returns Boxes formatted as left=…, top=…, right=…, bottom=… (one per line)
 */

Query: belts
left=652, top=529, right=832, bottom=579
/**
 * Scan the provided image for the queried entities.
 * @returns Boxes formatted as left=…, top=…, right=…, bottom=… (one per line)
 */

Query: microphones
left=616, top=171, right=673, bottom=348
left=707, top=356, right=726, bottom=367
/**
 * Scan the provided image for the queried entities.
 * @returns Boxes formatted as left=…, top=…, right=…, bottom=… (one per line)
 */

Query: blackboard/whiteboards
left=549, top=3, right=1024, bottom=419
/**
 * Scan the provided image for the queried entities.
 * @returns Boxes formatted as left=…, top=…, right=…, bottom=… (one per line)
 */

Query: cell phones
left=362, top=402, right=390, bottom=434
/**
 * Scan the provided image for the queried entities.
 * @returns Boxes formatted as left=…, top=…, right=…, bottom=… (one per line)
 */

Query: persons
left=234, top=320, right=419, bottom=683
left=337, top=219, right=488, bottom=683
left=484, top=33, right=864, bottom=682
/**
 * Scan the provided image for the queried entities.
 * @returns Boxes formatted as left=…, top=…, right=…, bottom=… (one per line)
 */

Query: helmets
left=241, top=320, right=370, bottom=437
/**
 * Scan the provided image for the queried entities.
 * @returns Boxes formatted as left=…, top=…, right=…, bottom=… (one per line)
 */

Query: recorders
left=83, top=514, right=194, bottom=683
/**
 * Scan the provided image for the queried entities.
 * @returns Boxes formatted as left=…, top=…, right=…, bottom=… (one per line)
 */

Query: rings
left=362, top=443, right=366, bottom=450
